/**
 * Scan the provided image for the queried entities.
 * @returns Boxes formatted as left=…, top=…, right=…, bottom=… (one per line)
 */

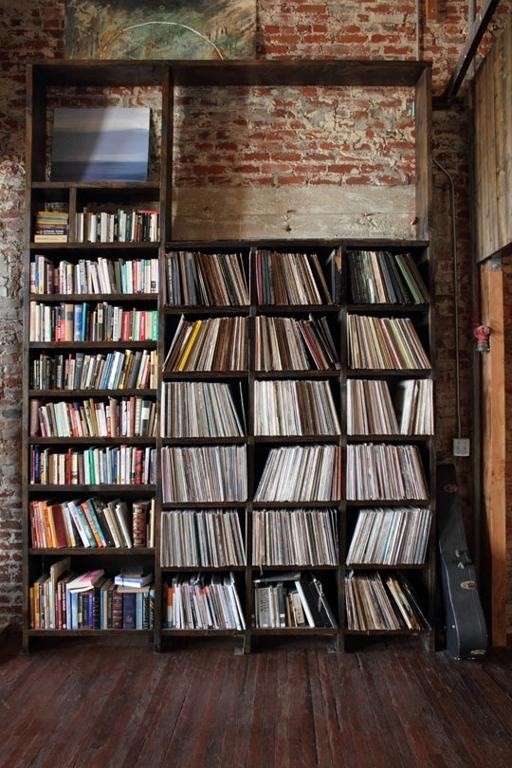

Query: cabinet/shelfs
left=21, top=61, right=439, bottom=656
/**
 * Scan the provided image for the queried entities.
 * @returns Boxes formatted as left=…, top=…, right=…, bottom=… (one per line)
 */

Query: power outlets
left=453, top=439, right=469, bottom=456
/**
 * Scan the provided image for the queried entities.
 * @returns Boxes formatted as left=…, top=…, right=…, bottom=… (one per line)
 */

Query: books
left=347, top=375, right=434, bottom=437
left=253, top=448, right=338, bottom=500
left=163, top=574, right=245, bottom=631
left=162, top=314, right=247, bottom=370
left=255, top=247, right=336, bottom=304
left=29, top=558, right=154, bottom=632
left=161, top=511, right=246, bottom=568
left=251, top=509, right=337, bottom=565
left=31, top=444, right=156, bottom=486
left=34, top=200, right=67, bottom=242
left=349, top=248, right=433, bottom=306
left=27, top=496, right=154, bottom=548
left=254, top=310, right=341, bottom=370
left=30, top=251, right=159, bottom=294
left=31, top=397, right=158, bottom=437
left=32, top=351, right=158, bottom=392
left=345, top=506, right=432, bottom=565
left=253, top=574, right=335, bottom=626
left=348, top=311, right=431, bottom=370
left=29, top=302, right=159, bottom=340
left=160, top=446, right=246, bottom=504
left=162, top=381, right=246, bottom=438
left=344, top=573, right=428, bottom=631
left=77, top=210, right=159, bottom=243
left=346, top=443, right=430, bottom=499
left=253, top=378, right=342, bottom=435
left=166, top=250, right=252, bottom=307
